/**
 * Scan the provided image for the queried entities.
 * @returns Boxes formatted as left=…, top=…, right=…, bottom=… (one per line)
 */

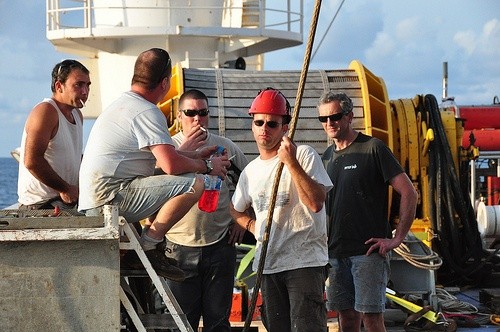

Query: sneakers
left=124, top=236, right=185, bottom=281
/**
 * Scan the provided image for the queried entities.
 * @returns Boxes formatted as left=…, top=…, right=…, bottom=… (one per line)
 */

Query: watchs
left=202, top=159, right=213, bottom=174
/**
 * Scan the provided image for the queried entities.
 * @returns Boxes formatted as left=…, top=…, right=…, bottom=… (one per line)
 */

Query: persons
left=229, top=87, right=334, bottom=332
left=78, top=48, right=232, bottom=282
left=316, top=91, right=418, bottom=332
left=153, top=89, right=249, bottom=332
left=17, top=59, right=91, bottom=209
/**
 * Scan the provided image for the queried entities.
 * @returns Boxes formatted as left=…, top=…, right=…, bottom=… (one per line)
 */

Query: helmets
left=248, top=87, right=291, bottom=115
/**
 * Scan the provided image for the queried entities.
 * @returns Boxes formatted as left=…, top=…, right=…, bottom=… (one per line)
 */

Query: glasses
left=58, top=60, right=77, bottom=81
left=180, top=109, right=209, bottom=117
left=159, top=49, right=170, bottom=83
left=253, top=120, right=285, bottom=128
left=318, top=112, right=348, bottom=122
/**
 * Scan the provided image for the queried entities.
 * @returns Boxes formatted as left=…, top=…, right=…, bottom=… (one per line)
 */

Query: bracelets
left=246, top=219, right=254, bottom=231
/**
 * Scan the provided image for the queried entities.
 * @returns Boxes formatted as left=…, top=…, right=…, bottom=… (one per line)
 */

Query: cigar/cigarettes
left=228, top=153, right=236, bottom=160
left=79, top=99, right=86, bottom=107
left=199, top=127, right=206, bottom=131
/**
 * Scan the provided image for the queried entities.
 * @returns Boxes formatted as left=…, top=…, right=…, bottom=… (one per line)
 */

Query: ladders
left=119, top=216, right=194, bottom=332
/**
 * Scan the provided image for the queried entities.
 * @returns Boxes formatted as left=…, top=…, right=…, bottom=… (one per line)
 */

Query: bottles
left=197, top=146, right=229, bottom=213
